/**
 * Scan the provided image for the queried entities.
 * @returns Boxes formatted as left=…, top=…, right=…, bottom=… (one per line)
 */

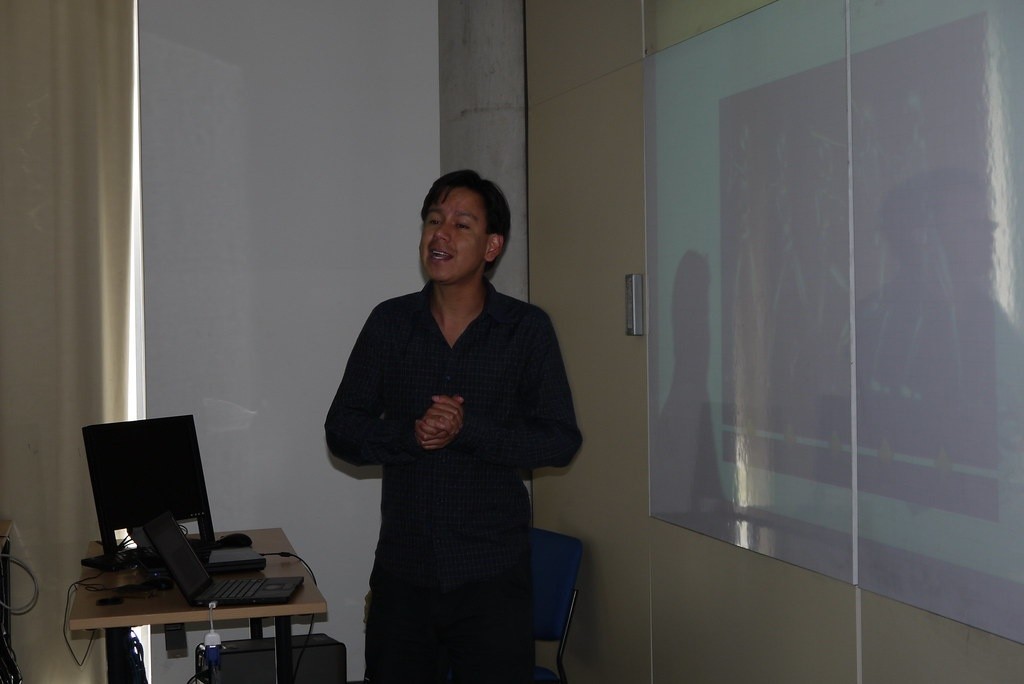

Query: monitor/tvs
left=82, top=414, right=215, bottom=573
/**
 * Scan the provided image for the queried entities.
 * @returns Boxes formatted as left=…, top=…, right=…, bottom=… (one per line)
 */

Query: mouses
left=214, top=533, right=253, bottom=548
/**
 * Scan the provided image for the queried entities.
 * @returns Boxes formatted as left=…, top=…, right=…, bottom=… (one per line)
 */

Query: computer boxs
left=194, top=633, right=348, bottom=684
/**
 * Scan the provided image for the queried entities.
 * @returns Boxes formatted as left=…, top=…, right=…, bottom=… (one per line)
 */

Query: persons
left=324, top=169, right=583, bottom=684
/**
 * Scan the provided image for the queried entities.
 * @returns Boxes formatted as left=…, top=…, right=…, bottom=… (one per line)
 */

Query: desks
left=0, top=519, right=13, bottom=657
left=69, top=528, right=327, bottom=684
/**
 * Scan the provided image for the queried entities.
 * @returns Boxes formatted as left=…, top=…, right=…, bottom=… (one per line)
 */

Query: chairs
left=531, top=527, right=582, bottom=684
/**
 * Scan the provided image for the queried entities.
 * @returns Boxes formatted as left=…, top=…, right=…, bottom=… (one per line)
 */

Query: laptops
left=136, top=547, right=267, bottom=577
left=141, top=511, right=304, bottom=606
